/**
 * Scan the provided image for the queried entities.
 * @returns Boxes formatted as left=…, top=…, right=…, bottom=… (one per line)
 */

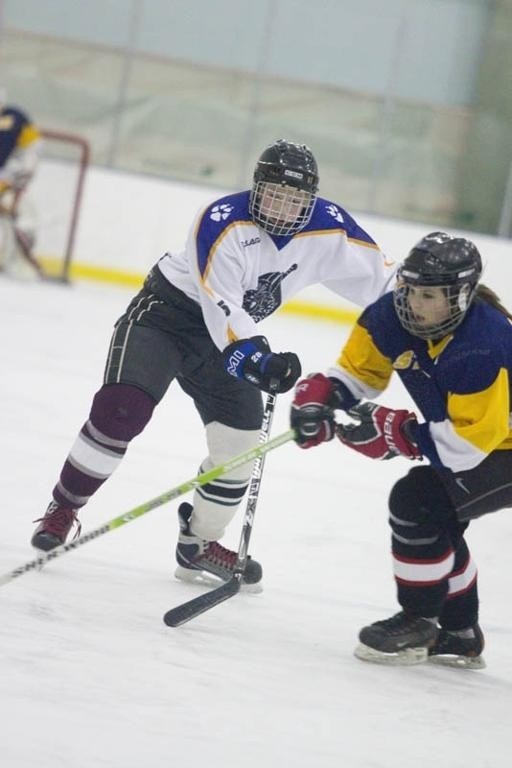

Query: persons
left=1, top=106, right=42, bottom=268
left=33, top=140, right=399, bottom=583
left=290, top=232, right=512, bottom=658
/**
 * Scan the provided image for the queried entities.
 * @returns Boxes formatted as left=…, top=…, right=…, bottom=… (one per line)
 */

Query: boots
left=174, top=502, right=264, bottom=586
left=29, top=491, right=82, bottom=553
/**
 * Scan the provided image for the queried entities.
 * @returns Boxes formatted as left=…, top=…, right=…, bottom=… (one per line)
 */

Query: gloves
left=333, top=401, right=425, bottom=464
left=218, top=335, right=302, bottom=394
left=289, top=370, right=357, bottom=450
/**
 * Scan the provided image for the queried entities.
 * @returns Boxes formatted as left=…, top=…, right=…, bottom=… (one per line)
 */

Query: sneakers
left=425, top=622, right=487, bottom=660
left=358, top=604, right=443, bottom=653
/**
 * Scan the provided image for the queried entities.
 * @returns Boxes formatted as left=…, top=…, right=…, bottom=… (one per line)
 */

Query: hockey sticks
left=165, top=377, right=279, bottom=629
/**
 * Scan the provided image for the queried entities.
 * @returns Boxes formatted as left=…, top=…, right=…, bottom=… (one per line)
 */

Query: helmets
left=247, top=139, right=321, bottom=210
left=399, top=232, right=482, bottom=315
